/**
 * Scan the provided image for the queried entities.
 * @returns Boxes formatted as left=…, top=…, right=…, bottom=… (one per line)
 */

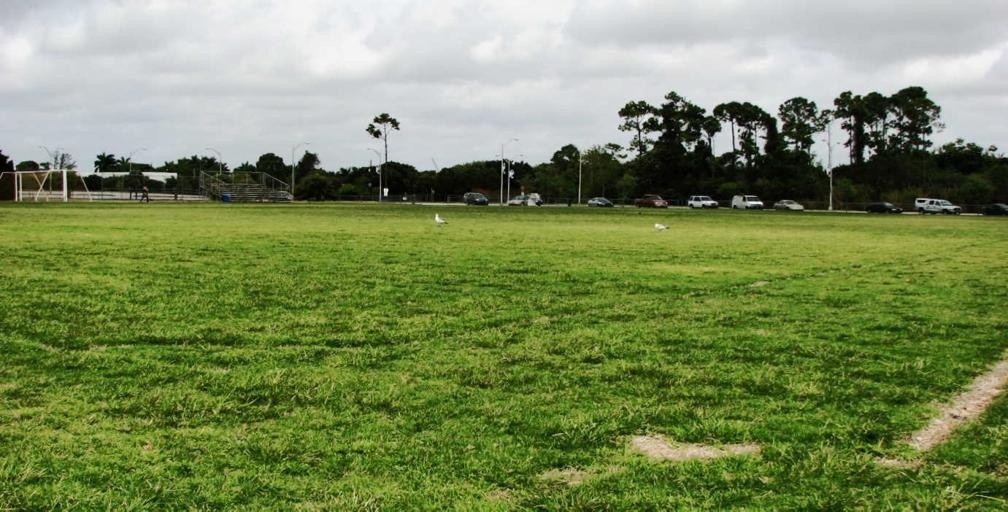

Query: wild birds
left=654, top=223, right=670, bottom=231
left=435, top=213, right=448, bottom=224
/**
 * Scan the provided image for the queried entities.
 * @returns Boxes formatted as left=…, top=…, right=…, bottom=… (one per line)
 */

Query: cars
left=509, top=195, right=544, bottom=206
left=978, top=197, right=1008, bottom=216
left=865, top=201, right=904, bottom=214
left=634, top=194, right=668, bottom=208
left=464, top=192, right=489, bottom=205
left=774, top=199, right=805, bottom=211
left=587, top=197, right=613, bottom=207
left=688, top=195, right=719, bottom=209
left=731, top=194, right=764, bottom=211
left=914, top=198, right=962, bottom=216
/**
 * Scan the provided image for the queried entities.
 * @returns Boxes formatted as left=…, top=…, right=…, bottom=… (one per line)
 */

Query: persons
left=139, top=184, right=150, bottom=203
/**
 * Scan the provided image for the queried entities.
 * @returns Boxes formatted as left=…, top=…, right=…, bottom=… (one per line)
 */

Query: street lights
left=500, top=136, right=523, bottom=206
left=292, top=141, right=310, bottom=196
left=204, top=147, right=222, bottom=175
left=366, top=147, right=382, bottom=202
